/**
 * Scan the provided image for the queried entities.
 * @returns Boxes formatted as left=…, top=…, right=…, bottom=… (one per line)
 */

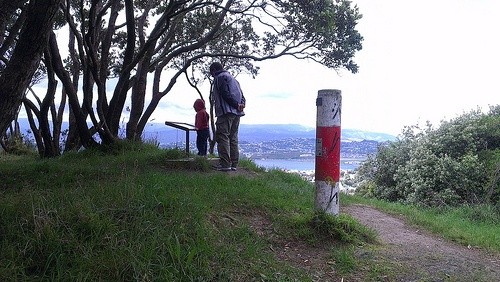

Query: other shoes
left=218, top=163, right=237, bottom=170
left=197, top=154, right=207, bottom=157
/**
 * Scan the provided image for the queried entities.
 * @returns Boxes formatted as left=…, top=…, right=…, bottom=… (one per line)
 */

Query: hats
left=194, top=99, right=204, bottom=111
left=209, top=62, right=226, bottom=74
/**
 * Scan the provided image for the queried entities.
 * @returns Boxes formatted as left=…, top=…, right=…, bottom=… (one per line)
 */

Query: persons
left=193, top=99, right=209, bottom=158
left=209, top=62, right=247, bottom=172
